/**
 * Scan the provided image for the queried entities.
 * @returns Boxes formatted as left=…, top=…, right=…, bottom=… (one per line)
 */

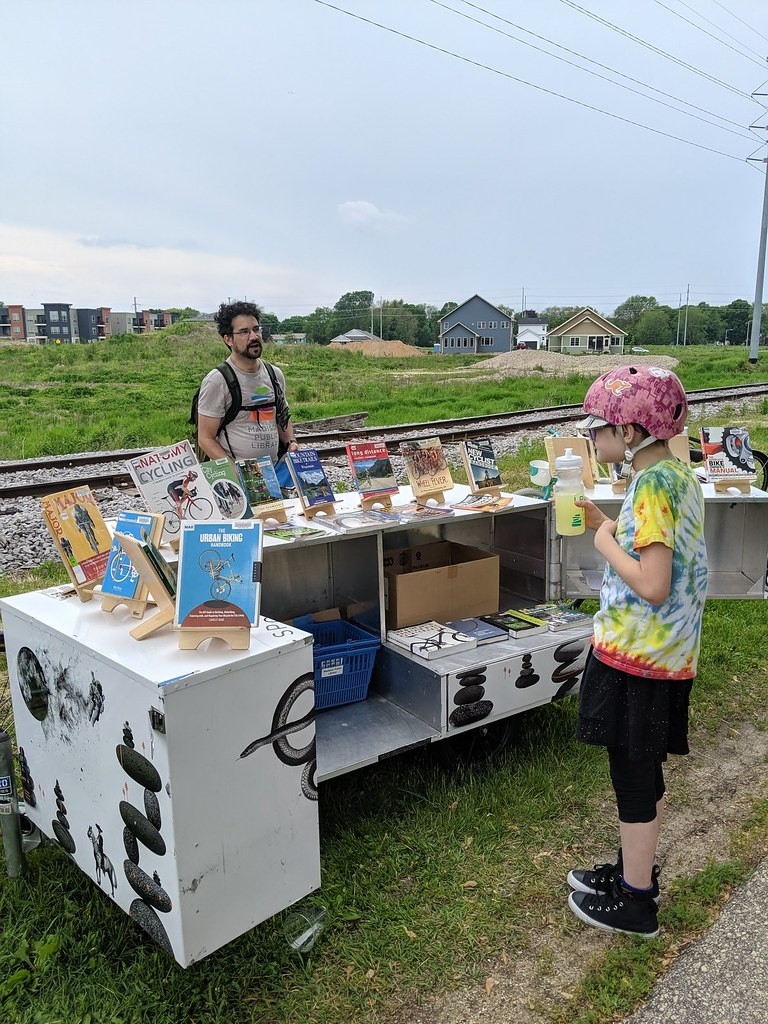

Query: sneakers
left=567, top=877, right=659, bottom=939
left=567, top=848, right=661, bottom=904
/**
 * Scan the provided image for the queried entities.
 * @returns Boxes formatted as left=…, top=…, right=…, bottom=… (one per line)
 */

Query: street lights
left=725, top=329, right=733, bottom=340
left=746, top=320, right=753, bottom=347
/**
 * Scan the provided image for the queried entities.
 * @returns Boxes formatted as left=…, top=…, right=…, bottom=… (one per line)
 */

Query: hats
left=576, top=416, right=610, bottom=430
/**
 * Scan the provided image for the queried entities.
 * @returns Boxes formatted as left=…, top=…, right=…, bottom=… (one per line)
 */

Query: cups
left=283, top=905, right=329, bottom=951
left=530, top=461, right=552, bottom=487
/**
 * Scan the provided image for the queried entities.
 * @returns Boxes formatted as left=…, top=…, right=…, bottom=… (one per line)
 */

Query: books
left=125, top=439, right=223, bottom=544
left=450, top=438, right=514, bottom=513
left=587, top=426, right=757, bottom=483
left=287, top=442, right=399, bottom=535
left=41, top=486, right=326, bottom=628
left=200, top=456, right=254, bottom=519
left=370, top=502, right=455, bottom=523
left=386, top=601, right=593, bottom=660
left=400, top=437, right=454, bottom=496
left=235, top=456, right=284, bottom=514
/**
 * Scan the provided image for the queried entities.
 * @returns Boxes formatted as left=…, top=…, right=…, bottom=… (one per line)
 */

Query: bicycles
left=689, top=436, right=768, bottom=492
left=411, top=448, right=447, bottom=480
left=162, top=487, right=213, bottom=534
left=110, top=531, right=139, bottom=584
left=79, top=521, right=100, bottom=555
left=199, top=550, right=243, bottom=602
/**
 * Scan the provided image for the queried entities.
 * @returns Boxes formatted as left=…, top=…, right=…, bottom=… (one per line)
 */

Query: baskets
left=292, top=614, right=381, bottom=710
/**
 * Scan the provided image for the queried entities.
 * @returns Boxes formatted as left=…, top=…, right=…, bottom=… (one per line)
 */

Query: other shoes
left=95, top=540, right=99, bottom=545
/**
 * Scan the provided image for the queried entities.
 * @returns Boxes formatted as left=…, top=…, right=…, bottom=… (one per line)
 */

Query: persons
left=213, top=482, right=241, bottom=515
left=407, top=447, right=443, bottom=474
left=60, top=537, right=73, bottom=558
left=198, top=301, right=299, bottom=480
left=74, top=504, right=99, bottom=550
left=322, top=486, right=329, bottom=497
left=485, top=470, right=491, bottom=486
left=243, top=461, right=267, bottom=493
left=365, top=469, right=371, bottom=484
left=551, top=365, right=708, bottom=938
left=336, top=517, right=380, bottom=528
left=167, top=471, right=198, bottom=522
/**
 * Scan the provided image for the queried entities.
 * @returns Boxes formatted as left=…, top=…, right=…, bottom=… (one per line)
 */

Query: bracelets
left=286, top=440, right=299, bottom=452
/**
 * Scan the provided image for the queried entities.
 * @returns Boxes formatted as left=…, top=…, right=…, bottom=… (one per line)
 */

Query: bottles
left=555, top=447, right=586, bottom=536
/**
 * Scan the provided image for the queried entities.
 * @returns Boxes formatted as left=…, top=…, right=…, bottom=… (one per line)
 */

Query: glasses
left=589, top=424, right=639, bottom=441
left=229, top=326, right=263, bottom=337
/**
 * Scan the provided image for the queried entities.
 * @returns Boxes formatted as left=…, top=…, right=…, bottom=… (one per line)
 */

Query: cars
left=627, top=347, right=650, bottom=353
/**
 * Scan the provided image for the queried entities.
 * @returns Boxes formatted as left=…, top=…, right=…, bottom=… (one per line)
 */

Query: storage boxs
left=294, top=619, right=381, bottom=710
left=383, top=540, right=501, bottom=630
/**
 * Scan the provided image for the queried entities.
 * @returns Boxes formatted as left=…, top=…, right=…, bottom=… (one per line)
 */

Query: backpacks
left=188, top=359, right=283, bottom=464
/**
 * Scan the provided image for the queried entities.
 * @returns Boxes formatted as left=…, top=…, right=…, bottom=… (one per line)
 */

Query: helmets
left=187, top=470, right=198, bottom=477
left=581, top=364, right=687, bottom=439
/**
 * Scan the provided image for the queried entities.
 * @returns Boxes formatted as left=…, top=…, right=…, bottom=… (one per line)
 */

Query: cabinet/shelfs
left=0, top=469, right=768, bottom=969
